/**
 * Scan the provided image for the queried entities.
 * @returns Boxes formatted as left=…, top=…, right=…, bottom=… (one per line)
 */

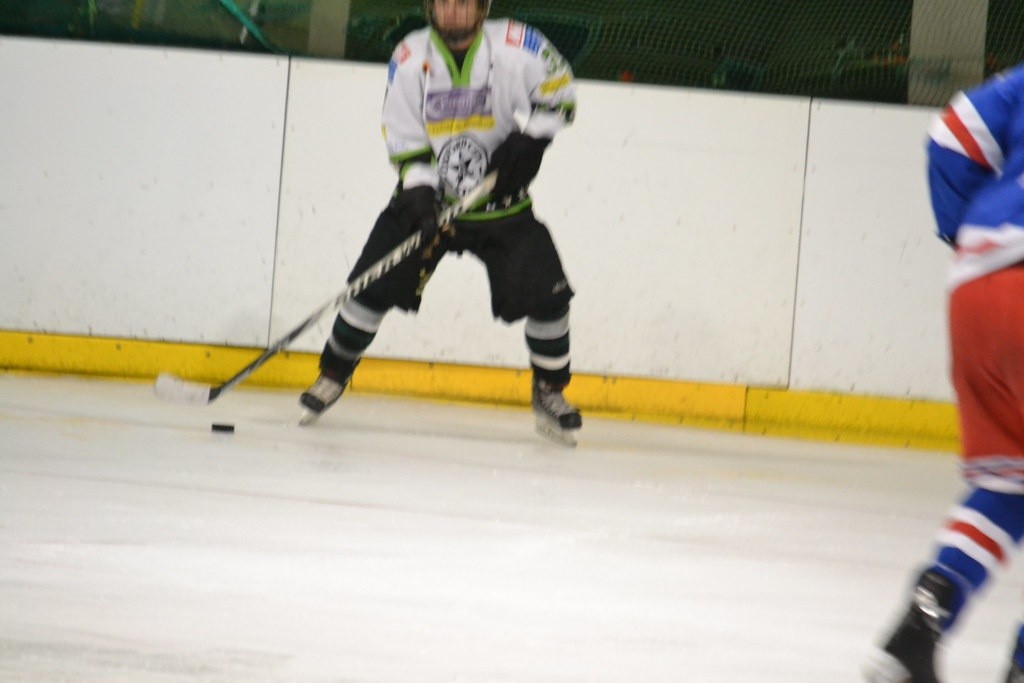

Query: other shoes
left=856, top=617, right=943, bottom=683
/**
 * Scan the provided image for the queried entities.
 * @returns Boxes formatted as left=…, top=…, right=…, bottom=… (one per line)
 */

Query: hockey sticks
left=154, top=170, right=497, bottom=409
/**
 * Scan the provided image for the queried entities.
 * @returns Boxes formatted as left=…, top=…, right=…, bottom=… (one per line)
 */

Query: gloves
left=484, top=134, right=553, bottom=203
left=398, top=186, right=440, bottom=261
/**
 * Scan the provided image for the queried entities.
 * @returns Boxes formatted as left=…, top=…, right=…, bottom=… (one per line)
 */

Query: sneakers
left=299, top=373, right=351, bottom=425
left=531, top=378, right=583, bottom=449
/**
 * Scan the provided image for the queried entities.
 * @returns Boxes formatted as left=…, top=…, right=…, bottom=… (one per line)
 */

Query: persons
left=861, top=58, right=1024, bottom=683
left=295, top=2, right=583, bottom=431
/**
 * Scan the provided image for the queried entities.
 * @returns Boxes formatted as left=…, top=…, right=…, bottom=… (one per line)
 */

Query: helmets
left=425, top=0, right=491, bottom=43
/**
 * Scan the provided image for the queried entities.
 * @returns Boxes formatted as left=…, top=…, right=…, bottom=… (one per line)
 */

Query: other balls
left=210, top=423, right=236, bottom=434
